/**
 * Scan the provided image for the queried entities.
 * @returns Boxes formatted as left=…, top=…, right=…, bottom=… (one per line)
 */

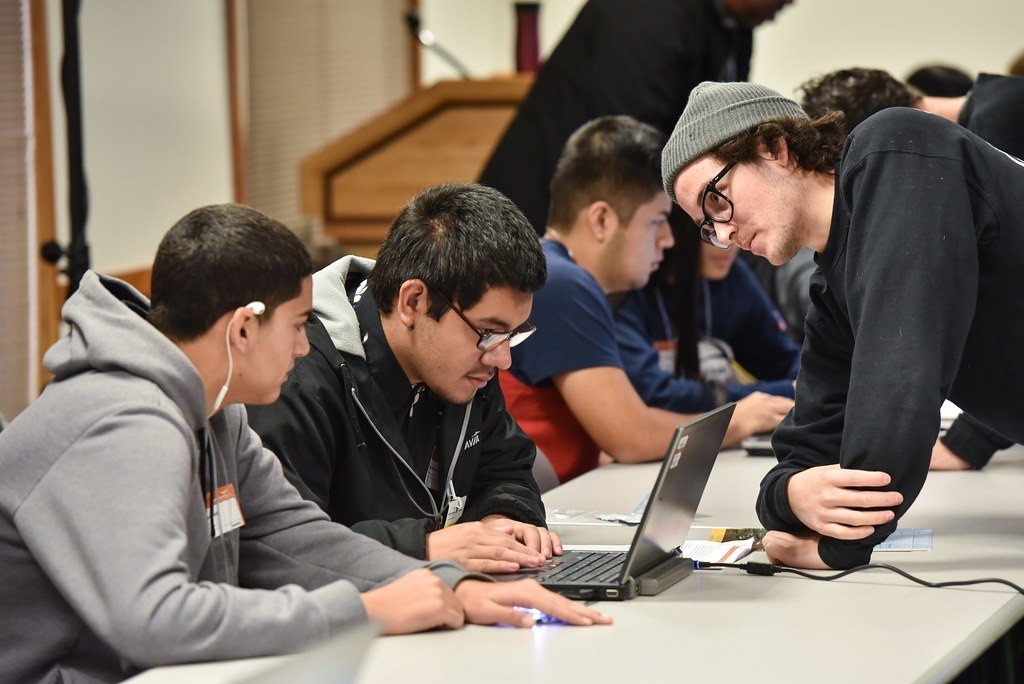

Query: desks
left=119, top=419, right=1024, bottom=684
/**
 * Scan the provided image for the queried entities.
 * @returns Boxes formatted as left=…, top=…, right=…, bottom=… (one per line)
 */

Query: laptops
left=484, top=401, right=738, bottom=601
left=741, top=432, right=775, bottom=457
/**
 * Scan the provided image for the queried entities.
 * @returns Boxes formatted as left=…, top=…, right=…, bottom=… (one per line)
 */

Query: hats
left=661, top=81, right=809, bottom=204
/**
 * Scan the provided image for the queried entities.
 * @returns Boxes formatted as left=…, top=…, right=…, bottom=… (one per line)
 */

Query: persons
left=611, top=197, right=801, bottom=416
left=477, top=0, right=798, bottom=284
left=791, top=66, right=1024, bottom=162
left=661, top=80, right=1024, bottom=570
left=241, top=182, right=563, bottom=577
left=495, top=113, right=797, bottom=488
left=0, top=202, right=614, bottom=684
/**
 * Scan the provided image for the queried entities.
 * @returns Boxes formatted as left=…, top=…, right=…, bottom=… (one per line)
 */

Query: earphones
left=248, top=301, right=266, bottom=315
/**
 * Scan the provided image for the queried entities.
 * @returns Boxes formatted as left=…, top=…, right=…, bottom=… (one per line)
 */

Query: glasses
left=432, top=287, right=537, bottom=352
left=700, top=160, right=740, bottom=250
